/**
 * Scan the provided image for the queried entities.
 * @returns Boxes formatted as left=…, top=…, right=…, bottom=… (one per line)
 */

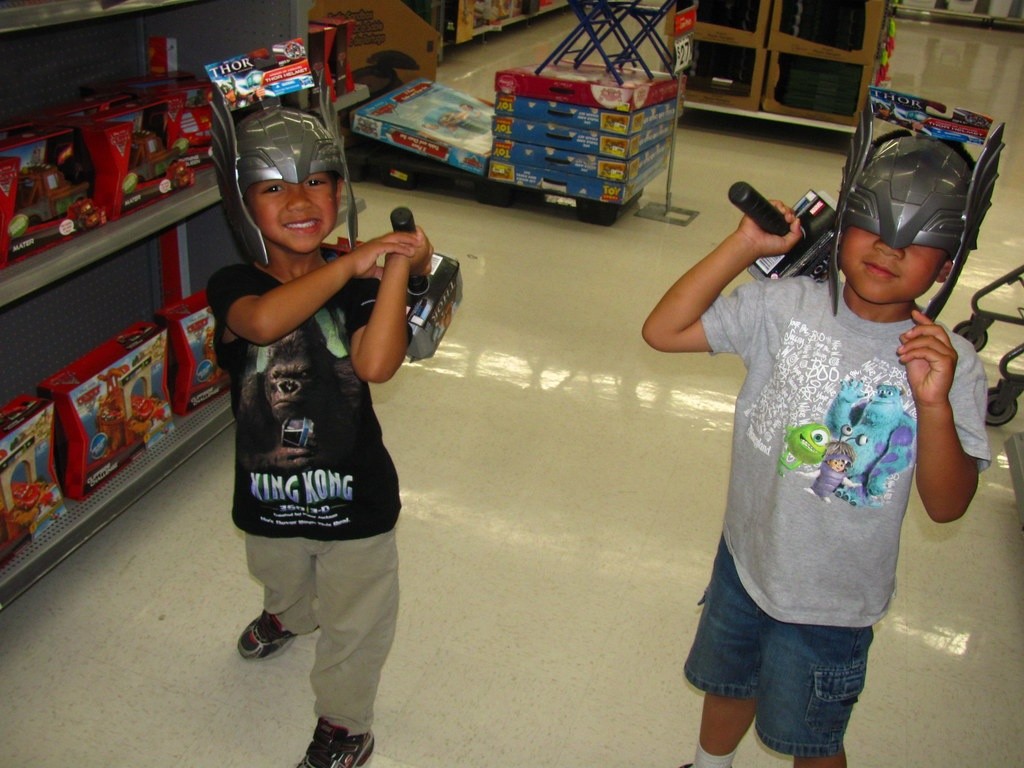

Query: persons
left=640, top=128, right=991, bottom=768
left=205, top=109, right=435, bottom=768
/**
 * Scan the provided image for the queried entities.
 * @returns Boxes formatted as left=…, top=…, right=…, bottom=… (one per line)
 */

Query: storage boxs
left=309, top=0, right=886, bottom=205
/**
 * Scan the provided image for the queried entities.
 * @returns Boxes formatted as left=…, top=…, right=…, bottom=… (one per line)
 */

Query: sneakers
left=295, top=718, right=375, bottom=768
left=237, top=608, right=320, bottom=661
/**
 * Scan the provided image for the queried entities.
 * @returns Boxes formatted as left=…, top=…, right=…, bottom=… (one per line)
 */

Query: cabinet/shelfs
left=0, top=0, right=306, bottom=609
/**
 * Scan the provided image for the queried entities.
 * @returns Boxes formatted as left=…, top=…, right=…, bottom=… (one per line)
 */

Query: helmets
left=828, top=88, right=1006, bottom=323
left=209, top=67, right=359, bottom=267
